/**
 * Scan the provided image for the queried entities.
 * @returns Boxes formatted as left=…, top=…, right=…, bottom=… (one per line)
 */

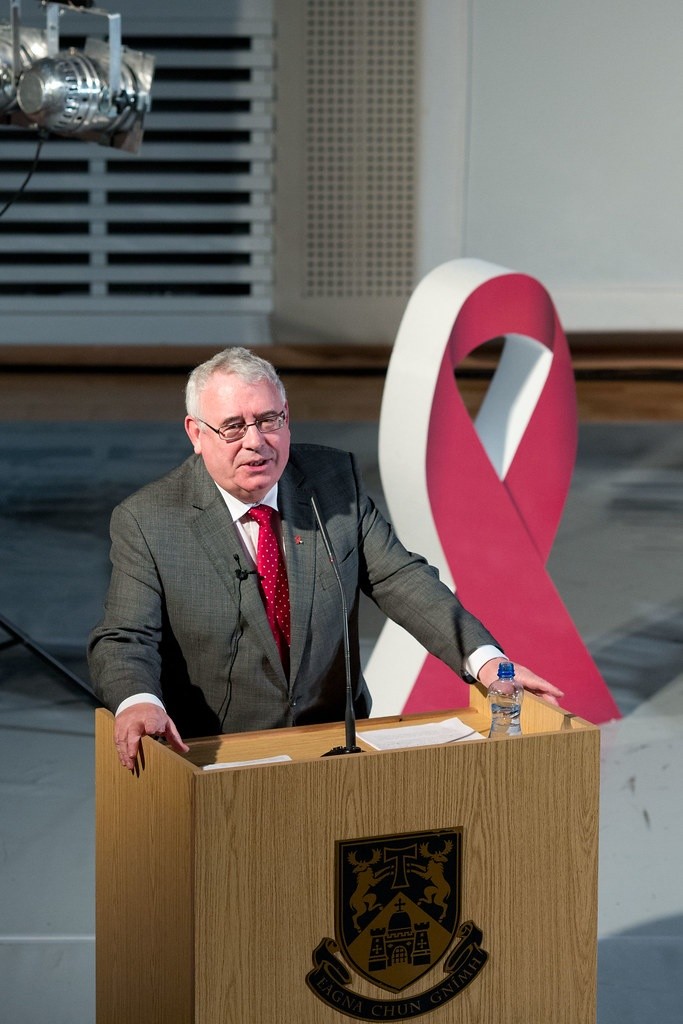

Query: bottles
left=487, top=663, right=524, bottom=738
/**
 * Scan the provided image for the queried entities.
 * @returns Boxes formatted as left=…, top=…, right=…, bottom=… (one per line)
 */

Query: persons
left=86, top=347, right=565, bottom=769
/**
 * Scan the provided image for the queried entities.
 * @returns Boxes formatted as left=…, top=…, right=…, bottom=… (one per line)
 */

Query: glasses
left=196, top=410, right=286, bottom=440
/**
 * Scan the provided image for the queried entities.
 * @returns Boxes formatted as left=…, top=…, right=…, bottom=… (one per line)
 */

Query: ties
left=247, top=504, right=291, bottom=677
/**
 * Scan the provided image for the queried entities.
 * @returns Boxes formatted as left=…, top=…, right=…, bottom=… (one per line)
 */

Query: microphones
left=232, top=554, right=248, bottom=581
left=308, top=490, right=366, bottom=757
